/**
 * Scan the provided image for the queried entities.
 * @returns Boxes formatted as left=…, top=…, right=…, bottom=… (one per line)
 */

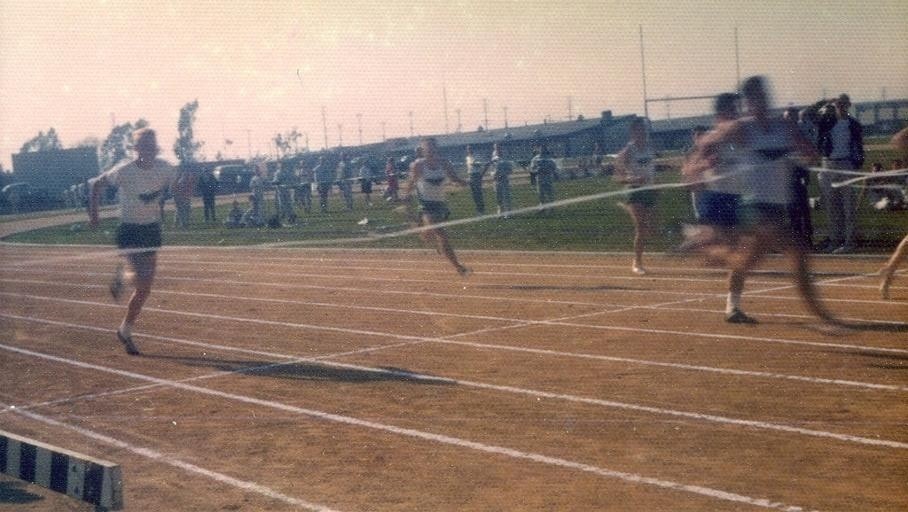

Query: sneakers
left=725, top=308, right=754, bottom=323
left=822, top=243, right=856, bottom=254
left=117, top=327, right=140, bottom=355
left=631, top=263, right=647, bottom=276
left=878, top=266, right=895, bottom=300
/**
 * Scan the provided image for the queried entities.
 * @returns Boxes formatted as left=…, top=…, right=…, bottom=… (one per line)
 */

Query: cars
left=0, top=182, right=48, bottom=207
left=213, top=165, right=255, bottom=187
